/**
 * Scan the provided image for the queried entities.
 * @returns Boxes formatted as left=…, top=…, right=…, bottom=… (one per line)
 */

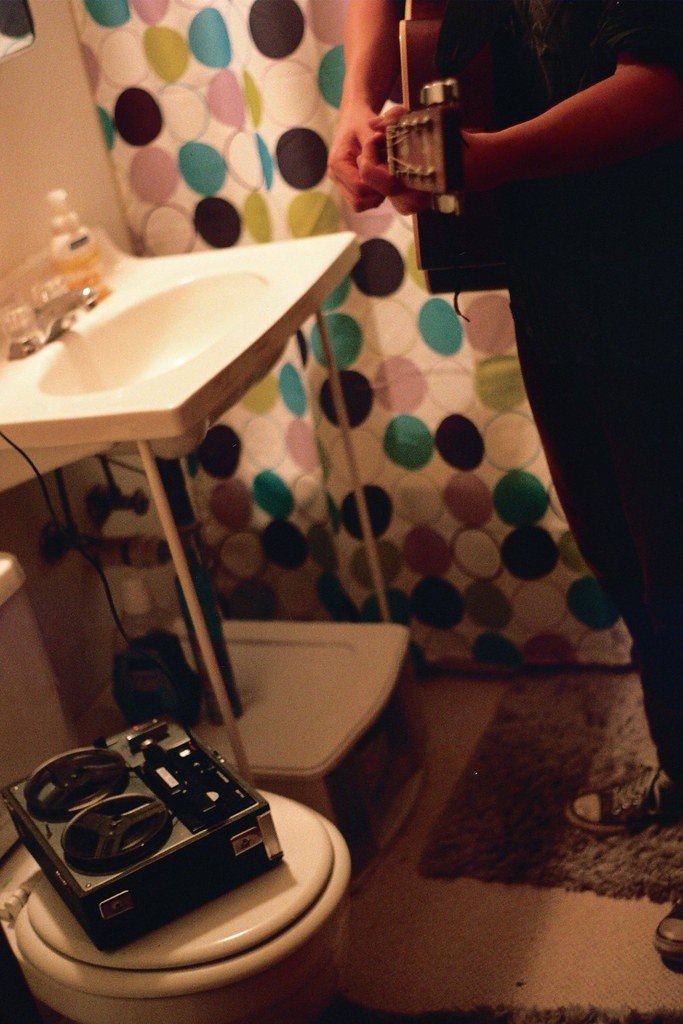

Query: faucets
left=6, top=287, right=97, bottom=362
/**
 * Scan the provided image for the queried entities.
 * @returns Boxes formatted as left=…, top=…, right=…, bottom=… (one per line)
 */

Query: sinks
left=2, top=231, right=365, bottom=447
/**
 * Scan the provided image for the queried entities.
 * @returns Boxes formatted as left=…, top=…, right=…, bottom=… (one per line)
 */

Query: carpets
left=420, top=664, right=683, bottom=906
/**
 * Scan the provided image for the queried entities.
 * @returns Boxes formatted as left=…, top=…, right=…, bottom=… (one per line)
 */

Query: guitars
left=382, top=0, right=466, bottom=218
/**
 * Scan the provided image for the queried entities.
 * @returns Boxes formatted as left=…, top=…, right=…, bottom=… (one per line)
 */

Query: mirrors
left=0, top=0, right=38, bottom=65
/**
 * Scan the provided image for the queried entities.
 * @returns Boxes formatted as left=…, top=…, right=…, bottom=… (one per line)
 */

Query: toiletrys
left=114, top=572, right=173, bottom=656
left=45, top=188, right=113, bottom=300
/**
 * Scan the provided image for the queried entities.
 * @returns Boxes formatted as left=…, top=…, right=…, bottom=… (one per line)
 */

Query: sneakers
left=653, top=899, right=683, bottom=963
left=567, top=762, right=681, bottom=832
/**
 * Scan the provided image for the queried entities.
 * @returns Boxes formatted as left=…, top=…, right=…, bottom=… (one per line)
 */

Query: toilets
left=0, top=553, right=353, bottom=1022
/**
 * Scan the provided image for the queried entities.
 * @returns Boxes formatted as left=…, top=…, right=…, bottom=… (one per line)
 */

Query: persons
left=328, top=0, right=683, bottom=965
